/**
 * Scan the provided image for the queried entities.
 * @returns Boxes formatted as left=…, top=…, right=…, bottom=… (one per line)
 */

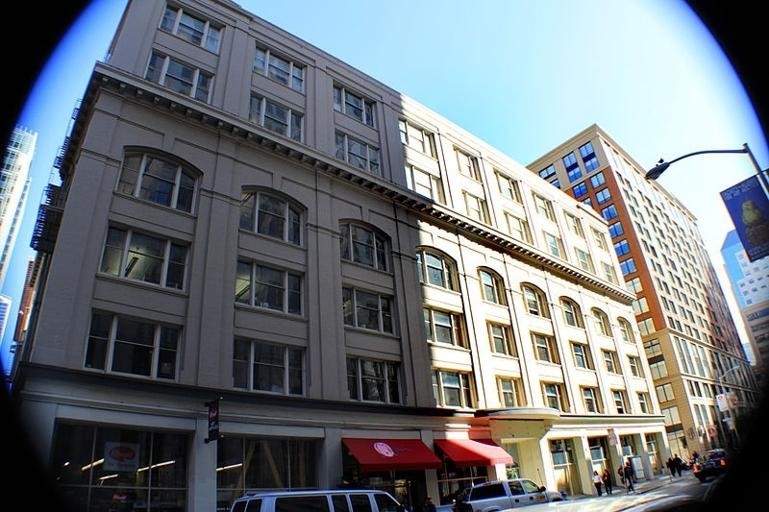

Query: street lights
left=719, top=364, right=740, bottom=440
left=644, top=140, right=769, bottom=199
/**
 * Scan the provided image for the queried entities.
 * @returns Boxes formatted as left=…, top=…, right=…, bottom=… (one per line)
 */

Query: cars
left=693, top=446, right=729, bottom=483
left=495, top=492, right=707, bottom=512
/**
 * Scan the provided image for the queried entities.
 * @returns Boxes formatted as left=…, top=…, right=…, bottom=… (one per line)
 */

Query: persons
left=667, top=457, right=676, bottom=478
left=424, top=495, right=439, bottom=512
left=450, top=489, right=473, bottom=511
left=617, top=466, right=628, bottom=489
left=592, top=470, right=604, bottom=497
left=673, top=453, right=683, bottom=477
left=624, top=461, right=638, bottom=493
left=602, top=469, right=613, bottom=496
left=692, top=450, right=700, bottom=462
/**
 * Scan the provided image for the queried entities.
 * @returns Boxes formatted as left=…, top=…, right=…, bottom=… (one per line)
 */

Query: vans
left=229, top=483, right=410, bottom=511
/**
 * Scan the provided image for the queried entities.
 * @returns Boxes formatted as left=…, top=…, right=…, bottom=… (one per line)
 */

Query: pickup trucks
left=432, top=478, right=565, bottom=511
